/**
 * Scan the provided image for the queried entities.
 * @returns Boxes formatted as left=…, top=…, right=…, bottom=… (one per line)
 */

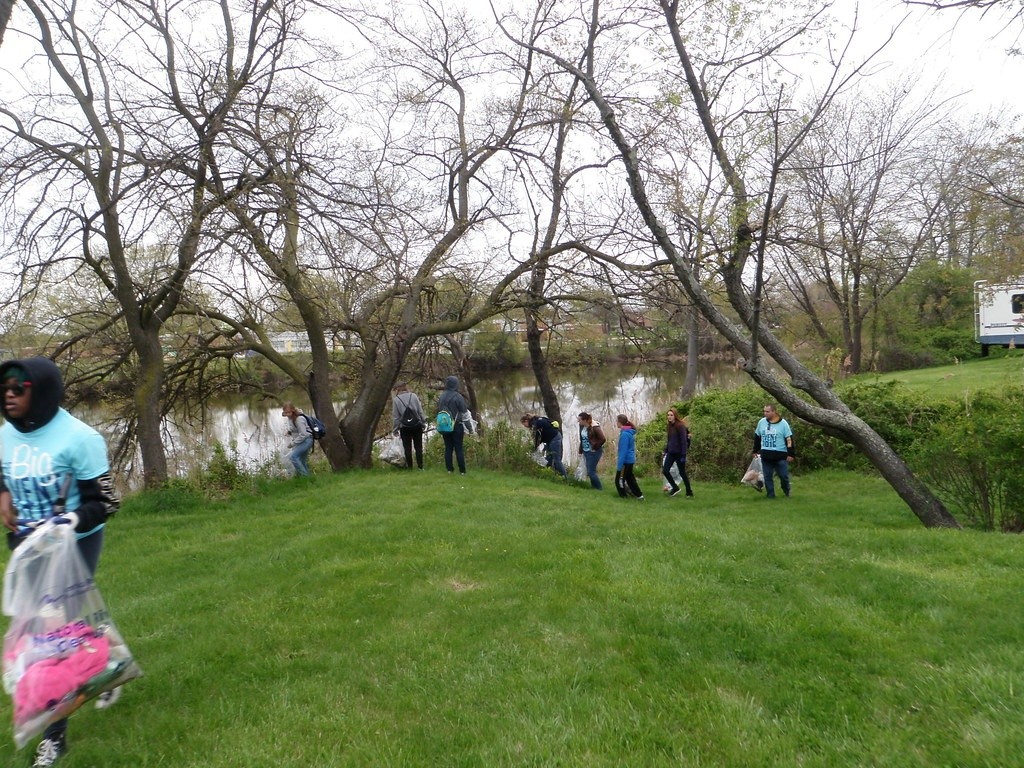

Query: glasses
left=2, top=381, right=30, bottom=396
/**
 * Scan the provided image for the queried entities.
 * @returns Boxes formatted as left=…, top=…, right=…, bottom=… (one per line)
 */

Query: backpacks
left=395, top=392, right=420, bottom=427
left=293, top=413, right=327, bottom=456
left=436, top=392, right=460, bottom=433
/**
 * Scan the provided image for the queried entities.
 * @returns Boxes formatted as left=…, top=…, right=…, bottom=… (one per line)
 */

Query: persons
left=0, top=357, right=120, bottom=768
left=520, top=413, right=567, bottom=482
left=439, top=376, right=474, bottom=476
left=661, top=407, right=693, bottom=498
left=614, top=415, right=643, bottom=500
left=393, top=381, right=426, bottom=470
left=752, top=403, right=794, bottom=499
left=577, top=412, right=606, bottom=491
left=282, top=401, right=314, bottom=479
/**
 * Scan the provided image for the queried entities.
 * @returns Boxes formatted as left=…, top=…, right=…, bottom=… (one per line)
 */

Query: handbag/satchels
left=741, top=454, right=765, bottom=493
left=2, top=510, right=145, bottom=751
left=661, top=451, right=682, bottom=492
left=576, top=454, right=587, bottom=481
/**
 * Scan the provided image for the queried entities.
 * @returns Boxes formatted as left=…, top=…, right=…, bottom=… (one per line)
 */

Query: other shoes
left=637, top=496, right=645, bottom=502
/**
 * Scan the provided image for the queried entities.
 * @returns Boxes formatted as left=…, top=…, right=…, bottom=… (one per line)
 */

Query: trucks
left=972, top=273, right=1024, bottom=359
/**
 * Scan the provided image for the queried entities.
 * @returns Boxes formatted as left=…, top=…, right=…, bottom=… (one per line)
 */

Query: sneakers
left=685, top=494, right=696, bottom=499
left=30, top=730, right=67, bottom=767
left=669, top=486, right=681, bottom=498
left=94, top=683, right=121, bottom=711
